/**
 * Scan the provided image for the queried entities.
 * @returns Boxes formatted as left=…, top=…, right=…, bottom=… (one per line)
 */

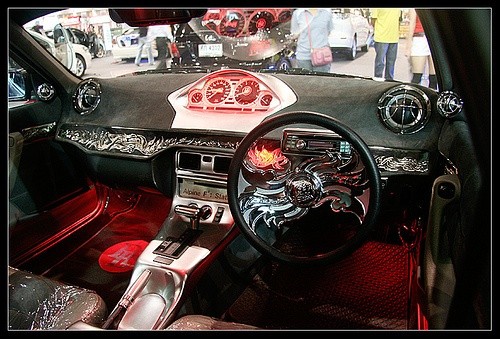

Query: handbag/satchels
left=310, top=47, right=333, bottom=67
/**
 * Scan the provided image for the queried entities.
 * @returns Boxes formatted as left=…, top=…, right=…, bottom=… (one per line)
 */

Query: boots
left=429, top=75, right=438, bottom=90
left=411, top=73, right=423, bottom=84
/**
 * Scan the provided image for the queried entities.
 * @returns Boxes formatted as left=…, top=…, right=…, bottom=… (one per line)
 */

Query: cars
left=111, top=26, right=172, bottom=60
left=328, top=12, right=372, bottom=61
left=7, top=22, right=105, bottom=102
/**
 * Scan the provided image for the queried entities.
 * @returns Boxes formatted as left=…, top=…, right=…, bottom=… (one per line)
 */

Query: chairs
left=8, top=266, right=107, bottom=330
left=162, top=315, right=260, bottom=331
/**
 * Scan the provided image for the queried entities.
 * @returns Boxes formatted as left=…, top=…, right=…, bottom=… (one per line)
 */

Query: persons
left=404, top=8, right=438, bottom=90
left=290, top=8, right=335, bottom=75
left=370, top=8, right=402, bottom=80
left=135, top=25, right=173, bottom=69
